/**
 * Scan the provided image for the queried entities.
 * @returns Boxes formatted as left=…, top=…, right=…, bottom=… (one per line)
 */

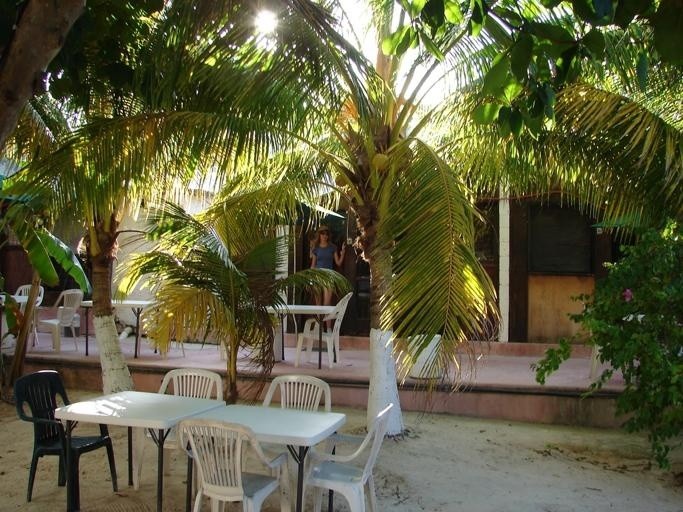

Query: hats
left=317, top=225, right=329, bottom=231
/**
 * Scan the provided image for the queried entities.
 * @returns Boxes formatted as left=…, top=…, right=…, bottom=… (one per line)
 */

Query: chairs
left=260, top=374, right=331, bottom=480
left=137, top=300, right=186, bottom=358
left=133, top=368, right=224, bottom=492
left=13, top=370, right=117, bottom=511
left=177, top=419, right=291, bottom=512
left=293, top=291, right=354, bottom=368
left=13, top=284, right=84, bottom=351
left=302, top=402, right=394, bottom=511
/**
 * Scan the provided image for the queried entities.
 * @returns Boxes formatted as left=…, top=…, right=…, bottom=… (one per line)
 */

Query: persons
left=307, top=224, right=348, bottom=334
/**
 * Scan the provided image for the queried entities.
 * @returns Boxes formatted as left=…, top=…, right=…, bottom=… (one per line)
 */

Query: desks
left=179, top=405, right=346, bottom=512
left=266, top=306, right=336, bottom=369
left=79, top=299, right=160, bottom=358
left=54, top=391, right=226, bottom=511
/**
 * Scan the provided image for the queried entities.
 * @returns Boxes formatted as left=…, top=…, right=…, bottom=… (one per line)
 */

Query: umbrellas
left=0, top=157, right=32, bottom=202
